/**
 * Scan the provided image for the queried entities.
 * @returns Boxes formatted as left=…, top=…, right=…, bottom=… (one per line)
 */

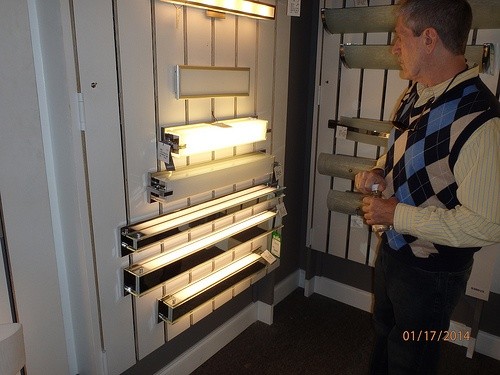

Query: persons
left=354, top=0, right=499, bottom=375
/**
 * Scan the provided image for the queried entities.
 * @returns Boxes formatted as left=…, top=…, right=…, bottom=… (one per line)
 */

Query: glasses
left=391, top=93, right=435, bottom=133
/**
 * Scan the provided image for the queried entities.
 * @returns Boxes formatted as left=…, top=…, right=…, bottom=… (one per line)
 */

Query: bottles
left=368, top=183, right=391, bottom=240
